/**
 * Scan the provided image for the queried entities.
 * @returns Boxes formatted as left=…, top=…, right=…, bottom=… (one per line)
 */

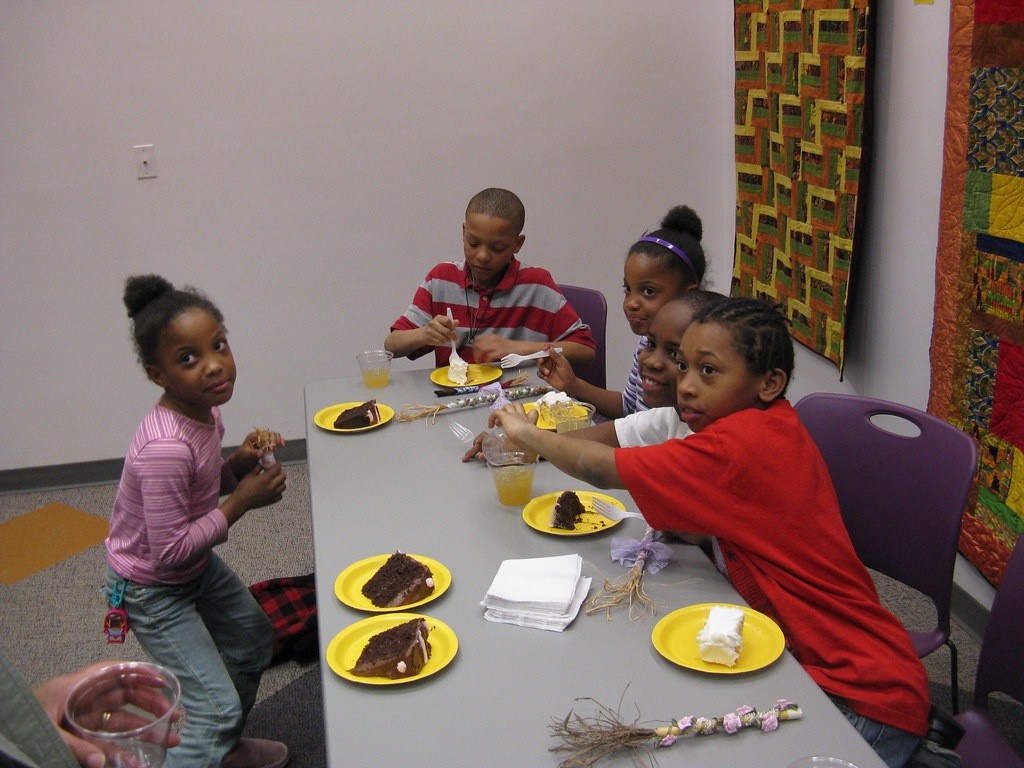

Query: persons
left=0, top=659, right=183, bottom=768
left=487, top=297, right=929, bottom=768
left=105, top=275, right=293, bottom=768
left=382, top=187, right=595, bottom=362
left=461, top=205, right=730, bottom=544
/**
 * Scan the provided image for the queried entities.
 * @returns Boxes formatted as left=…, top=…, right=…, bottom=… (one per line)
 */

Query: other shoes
left=924, top=704, right=967, bottom=751
left=220, top=737, right=289, bottom=768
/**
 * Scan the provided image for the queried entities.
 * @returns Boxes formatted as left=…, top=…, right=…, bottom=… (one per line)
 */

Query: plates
left=522, top=490, right=626, bottom=535
left=522, top=402, right=589, bottom=430
left=334, top=552, right=451, bottom=612
left=314, top=401, right=395, bottom=432
left=429, top=364, right=503, bottom=387
left=326, top=613, right=458, bottom=685
left=652, top=603, right=785, bottom=674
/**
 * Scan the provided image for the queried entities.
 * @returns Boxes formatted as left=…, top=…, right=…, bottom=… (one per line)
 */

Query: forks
left=448, top=421, right=475, bottom=443
left=446, top=308, right=460, bottom=366
left=501, top=348, right=563, bottom=369
left=592, top=497, right=647, bottom=522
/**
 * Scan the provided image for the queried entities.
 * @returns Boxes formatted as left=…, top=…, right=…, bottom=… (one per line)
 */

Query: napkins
left=481, top=553, right=591, bottom=633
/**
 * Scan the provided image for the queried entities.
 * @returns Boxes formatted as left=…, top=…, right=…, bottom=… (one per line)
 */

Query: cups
left=787, top=754, right=859, bottom=768
left=487, top=451, right=536, bottom=506
left=482, top=424, right=540, bottom=466
left=356, top=350, right=394, bottom=389
left=64, top=660, right=182, bottom=768
left=550, top=401, right=596, bottom=434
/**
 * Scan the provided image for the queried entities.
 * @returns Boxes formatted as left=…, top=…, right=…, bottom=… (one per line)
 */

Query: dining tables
left=304, top=359, right=890, bottom=768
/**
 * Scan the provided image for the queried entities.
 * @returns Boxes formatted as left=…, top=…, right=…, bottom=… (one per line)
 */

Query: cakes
left=536, top=390, right=572, bottom=423
left=447, top=359, right=471, bottom=385
left=549, top=490, right=585, bottom=531
left=696, top=605, right=746, bottom=668
left=352, top=617, right=432, bottom=678
left=361, top=552, right=434, bottom=608
left=334, top=400, right=380, bottom=429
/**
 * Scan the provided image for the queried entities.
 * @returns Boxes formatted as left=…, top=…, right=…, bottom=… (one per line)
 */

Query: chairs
left=948, top=537, right=1024, bottom=768
left=792, top=392, right=979, bottom=716
left=555, top=283, right=608, bottom=391
